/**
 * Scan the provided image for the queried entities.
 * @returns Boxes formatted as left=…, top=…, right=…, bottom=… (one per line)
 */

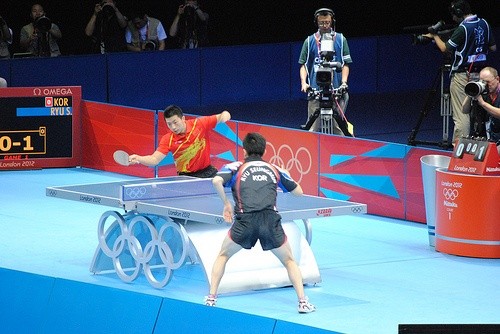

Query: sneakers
left=297, top=297, right=316, bottom=313
left=204, top=294, right=217, bottom=307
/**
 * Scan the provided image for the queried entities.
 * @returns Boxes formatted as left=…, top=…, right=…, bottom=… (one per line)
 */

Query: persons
left=85, top=0, right=128, bottom=54
left=167, top=0, right=209, bottom=49
left=129, top=104, right=232, bottom=178
left=14, top=3, right=61, bottom=56
left=204, top=133, right=316, bottom=313
left=124, top=7, right=167, bottom=52
left=297, top=9, right=352, bottom=134
left=462, top=66, right=500, bottom=149
left=0, top=16, right=13, bottom=58
left=421, top=0, right=490, bottom=149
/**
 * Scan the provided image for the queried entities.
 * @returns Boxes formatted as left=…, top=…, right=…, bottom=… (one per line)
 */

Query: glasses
left=316, top=22, right=333, bottom=27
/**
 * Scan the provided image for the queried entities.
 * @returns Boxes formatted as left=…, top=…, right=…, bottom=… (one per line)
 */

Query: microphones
left=404, top=25, right=430, bottom=33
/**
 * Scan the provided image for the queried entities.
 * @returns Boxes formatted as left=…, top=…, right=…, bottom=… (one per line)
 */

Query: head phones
left=450, top=2, right=464, bottom=19
left=313, top=8, right=336, bottom=27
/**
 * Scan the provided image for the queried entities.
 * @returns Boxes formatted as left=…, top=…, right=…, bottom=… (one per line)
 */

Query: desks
left=434, top=169, right=500, bottom=258
left=45, top=176, right=368, bottom=297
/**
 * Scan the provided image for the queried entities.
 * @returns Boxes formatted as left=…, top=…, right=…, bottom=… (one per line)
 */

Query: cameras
left=142, top=40, right=158, bottom=51
left=464, top=79, right=489, bottom=99
left=34, top=13, right=54, bottom=33
left=184, top=6, right=195, bottom=15
left=101, top=3, right=115, bottom=19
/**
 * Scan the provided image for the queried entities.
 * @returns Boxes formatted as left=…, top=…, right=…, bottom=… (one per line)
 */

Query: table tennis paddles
left=113, top=151, right=139, bottom=166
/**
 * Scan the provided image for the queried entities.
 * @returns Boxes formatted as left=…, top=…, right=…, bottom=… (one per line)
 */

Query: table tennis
left=152, top=183, right=157, bottom=188
